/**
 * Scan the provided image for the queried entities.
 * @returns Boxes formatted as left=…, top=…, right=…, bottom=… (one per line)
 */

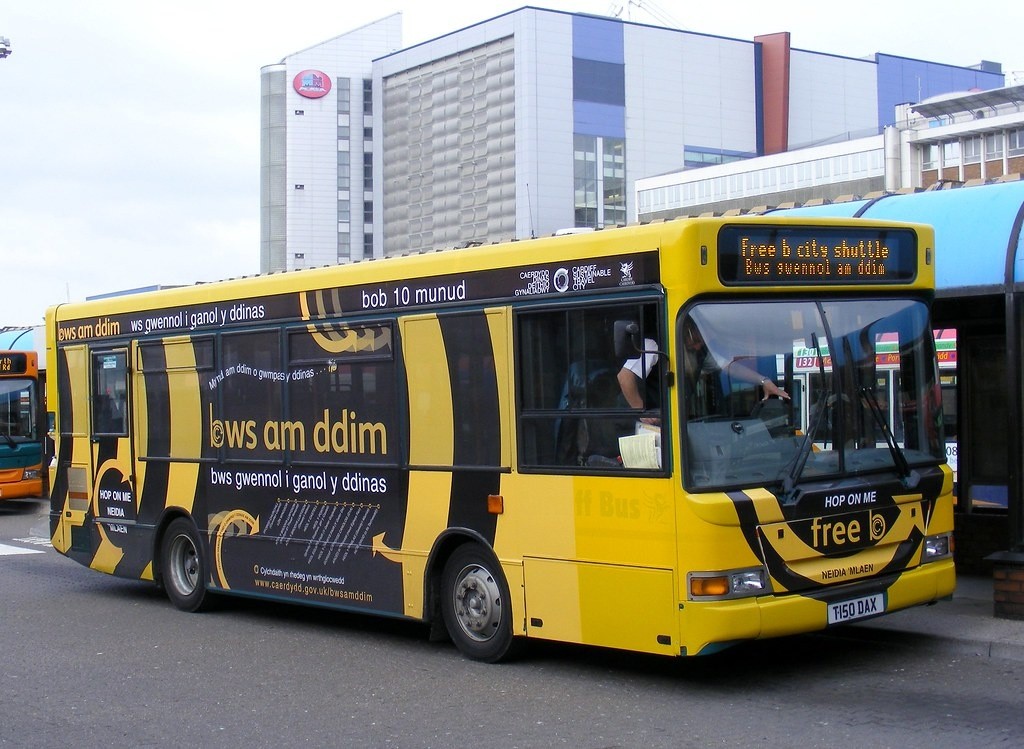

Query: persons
left=612, top=306, right=789, bottom=447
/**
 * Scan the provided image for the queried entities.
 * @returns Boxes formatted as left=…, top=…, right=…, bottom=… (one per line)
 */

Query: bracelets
left=760, top=377, right=771, bottom=386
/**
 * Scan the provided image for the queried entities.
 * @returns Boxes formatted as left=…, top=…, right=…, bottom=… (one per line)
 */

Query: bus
left=0, top=350, right=44, bottom=500
left=46, top=214, right=959, bottom=663
